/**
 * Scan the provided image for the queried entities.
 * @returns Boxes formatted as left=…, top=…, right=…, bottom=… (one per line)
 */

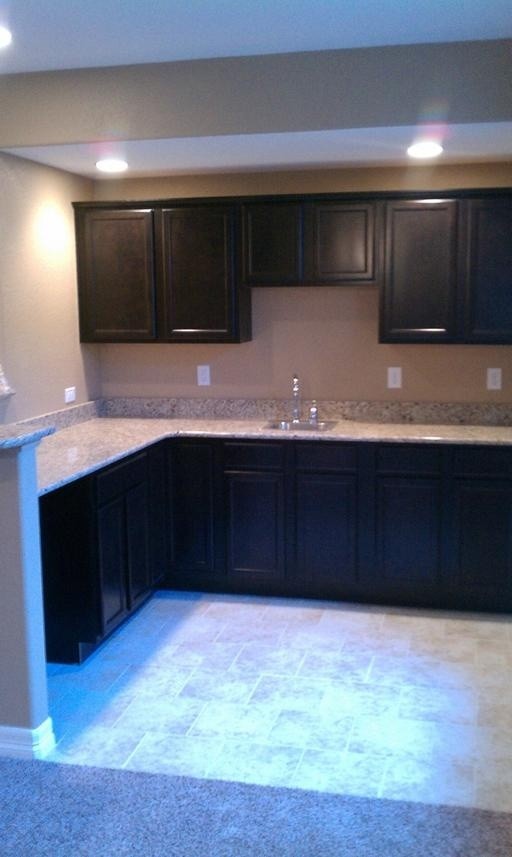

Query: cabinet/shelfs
left=74, top=200, right=244, bottom=348
left=168, top=431, right=511, bottom=615
left=39, top=438, right=167, bottom=657
left=241, top=195, right=378, bottom=285
left=378, top=189, right=512, bottom=345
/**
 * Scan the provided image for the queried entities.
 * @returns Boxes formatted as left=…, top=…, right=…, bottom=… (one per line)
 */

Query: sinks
left=260, top=419, right=339, bottom=432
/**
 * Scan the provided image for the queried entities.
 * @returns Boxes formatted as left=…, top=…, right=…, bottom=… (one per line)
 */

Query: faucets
left=290, top=373, right=301, bottom=421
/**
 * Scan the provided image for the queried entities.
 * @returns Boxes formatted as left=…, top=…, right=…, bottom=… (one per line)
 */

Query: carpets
left=0, top=755, right=512, bottom=857
